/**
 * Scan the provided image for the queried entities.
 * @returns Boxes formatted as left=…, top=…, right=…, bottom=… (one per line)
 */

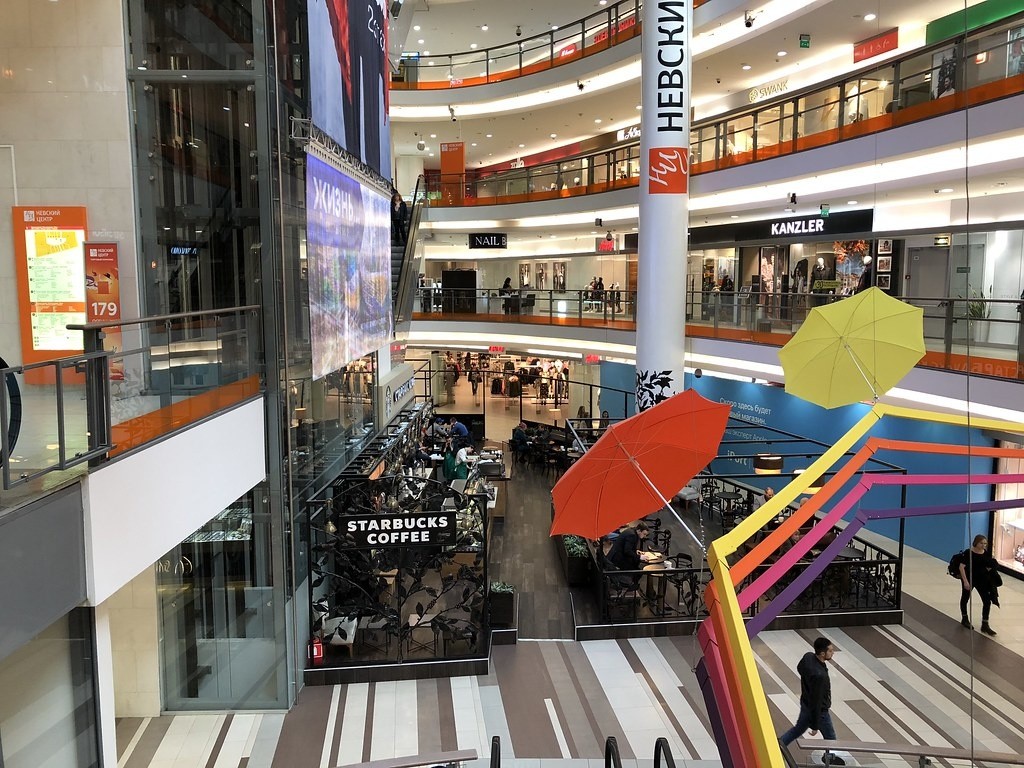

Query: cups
left=561, top=446, right=565, bottom=451
left=532, top=436, right=538, bottom=441
left=432, top=454, right=441, bottom=458
left=575, top=447, right=578, bottom=451
left=664, top=561, right=672, bottom=570
left=814, top=549, right=819, bottom=554
left=779, top=517, right=784, bottom=522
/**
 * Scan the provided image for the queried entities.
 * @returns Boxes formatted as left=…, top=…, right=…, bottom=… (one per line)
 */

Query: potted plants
left=550, top=502, right=590, bottom=586
left=957, top=282, right=993, bottom=342
left=488, top=581, right=516, bottom=625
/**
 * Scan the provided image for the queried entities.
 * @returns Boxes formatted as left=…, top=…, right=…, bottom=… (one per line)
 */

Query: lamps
left=784, top=193, right=798, bottom=214
left=594, top=218, right=603, bottom=227
left=705, top=217, right=708, bottom=223
left=448, top=105, right=457, bottom=122
left=515, top=24, right=522, bottom=37
left=745, top=9, right=764, bottom=28
left=382, top=403, right=436, bottom=476
left=606, top=231, right=613, bottom=241
left=577, top=79, right=584, bottom=90
left=416, top=135, right=425, bottom=151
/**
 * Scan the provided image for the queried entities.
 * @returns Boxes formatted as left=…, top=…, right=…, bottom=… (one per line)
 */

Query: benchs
left=798, top=532, right=837, bottom=552
left=762, top=526, right=812, bottom=535
left=314, top=617, right=357, bottom=659
left=745, top=543, right=782, bottom=563
left=676, top=486, right=700, bottom=513
left=593, top=540, right=641, bottom=623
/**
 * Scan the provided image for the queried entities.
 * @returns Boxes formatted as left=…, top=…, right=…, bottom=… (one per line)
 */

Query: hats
left=436, top=418, right=444, bottom=423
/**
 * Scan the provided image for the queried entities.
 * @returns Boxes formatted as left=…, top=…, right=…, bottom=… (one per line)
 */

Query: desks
left=488, top=480, right=506, bottom=526
left=407, top=613, right=439, bottom=657
left=640, top=551, right=667, bottom=607
left=836, top=547, right=865, bottom=598
left=566, top=452, right=584, bottom=458
left=481, top=454, right=497, bottom=459
left=547, top=441, right=555, bottom=445
left=359, top=616, right=392, bottom=656
left=441, top=425, right=450, bottom=430
left=643, top=564, right=672, bottom=617
left=714, top=492, right=743, bottom=527
left=435, top=438, right=447, bottom=443
left=483, top=445, right=499, bottom=454
left=619, top=520, right=648, bottom=534
left=551, top=446, right=563, bottom=452
left=404, top=467, right=433, bottom=500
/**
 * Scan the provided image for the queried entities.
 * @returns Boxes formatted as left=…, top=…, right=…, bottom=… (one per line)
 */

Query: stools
left=377, top=577, right=395, bottom=604
left=380, top=569, right=398, bottom=592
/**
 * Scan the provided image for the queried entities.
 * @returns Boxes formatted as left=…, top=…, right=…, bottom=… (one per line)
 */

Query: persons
left=726, top=138, right=734, bottom=156
left=720, top=275, right=733, bottom=296
left=809, top=258, right=836, bottom=305
left=780, top=638, right=834, bottom=746
left=1011, top=33, right=1022, bottom=75
left=502, top=277, right=512, bottom=313
left=761, top=254, right=805, bottom=319
left=465, top=187, right=472, bottom=199
left=858, top=257, right=873, bottom=292
left=690, top=151, right=695, bottom=164
left=391, top=191, right=407, bottom=247
left=958, top=535, right=998, bottom=636
left=584, top=275, right=623, bottom=314
left=940, top=75, right=955, bottom=98
left=551, top=172, right=581, bottom=190
left=414, top=351, right=814, bottom=584
left=620, top=171, right=627, bottom=179
left=520, top=264, right=566, bottom=293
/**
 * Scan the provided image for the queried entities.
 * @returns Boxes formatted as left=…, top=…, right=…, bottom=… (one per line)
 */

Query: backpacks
left=948, top=550, right=968, bottom=579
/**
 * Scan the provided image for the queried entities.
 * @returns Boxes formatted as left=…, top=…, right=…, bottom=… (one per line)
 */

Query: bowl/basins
left=467, top=456, right=480, bottom=460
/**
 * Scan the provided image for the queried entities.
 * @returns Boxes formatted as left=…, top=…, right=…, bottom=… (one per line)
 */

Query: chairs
left=508, top=439, right=574, bottom=484
left=701, top=484, right=721, bottom=521
left=721, top=512, right=735, bottom=536
left=647, top=529, right=672, bottom=556
left=640, top=518, right=661, bottom=552
left=665, top=553, right=693, bottom=606
left=521, top=294, right=536, bottom=317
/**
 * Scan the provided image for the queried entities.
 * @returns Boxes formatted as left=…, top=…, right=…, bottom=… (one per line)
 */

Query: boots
left=961, top=615, right=975, bottom=630
left=980, top=621, right=996, bottom=636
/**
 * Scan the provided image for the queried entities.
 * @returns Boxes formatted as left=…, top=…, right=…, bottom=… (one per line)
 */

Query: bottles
left=473, top=450, right=480, bottom=456
left=415, top=470, right=419, bottom=477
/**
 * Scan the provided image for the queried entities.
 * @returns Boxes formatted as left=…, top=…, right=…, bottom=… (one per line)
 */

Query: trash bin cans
left=811, top=750, right=856, bottom=766
left=758, top=319, right=771, bottom=332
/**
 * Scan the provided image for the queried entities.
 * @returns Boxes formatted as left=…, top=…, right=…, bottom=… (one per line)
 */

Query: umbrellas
left=779, top=286, right=926, bottom=409
left=549, top=387, right=733, bottom=552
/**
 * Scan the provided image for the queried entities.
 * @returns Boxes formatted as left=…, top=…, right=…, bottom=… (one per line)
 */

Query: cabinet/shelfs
left=441, top=479, right=493, bottom=586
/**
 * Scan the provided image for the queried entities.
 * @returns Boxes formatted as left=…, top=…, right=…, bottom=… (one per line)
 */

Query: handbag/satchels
left=477, top=375, right=482, bottom=382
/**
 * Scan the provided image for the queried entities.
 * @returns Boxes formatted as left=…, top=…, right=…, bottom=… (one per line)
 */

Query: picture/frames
left=876, top=274, right=891, bottom=290
left=876, top=256, right=892, bottom=273
left=878, top=238, right=893, bottom=255
left=418, top=278, right=434, bottom=288
